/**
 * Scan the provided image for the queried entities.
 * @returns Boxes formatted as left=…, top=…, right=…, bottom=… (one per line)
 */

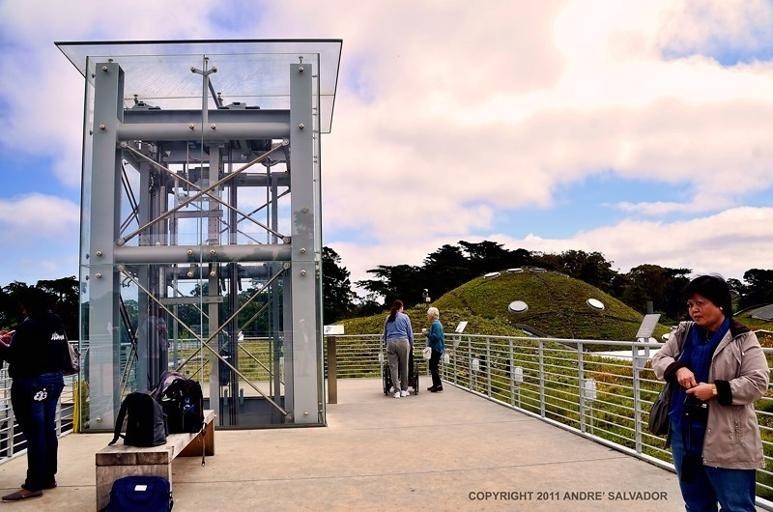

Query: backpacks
left=108, top=371, right=204, bottom=447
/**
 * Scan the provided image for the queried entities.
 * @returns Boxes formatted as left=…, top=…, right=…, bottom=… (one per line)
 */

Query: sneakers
left=392, top=390, right=410, bottom=398
left=428, top=384, right=442, bottom=392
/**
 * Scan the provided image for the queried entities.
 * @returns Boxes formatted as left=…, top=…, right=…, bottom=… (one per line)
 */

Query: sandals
left=1, top=481, right=57, bottom=502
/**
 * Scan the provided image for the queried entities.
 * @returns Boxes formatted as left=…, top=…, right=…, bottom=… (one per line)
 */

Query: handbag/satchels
left=98, top=476, right=173, bottom=512
left=422, top=347, right=432, bottom=360
left=64, top=332, right=81, bottom=376
left=649, top=383, right=671, bottom=436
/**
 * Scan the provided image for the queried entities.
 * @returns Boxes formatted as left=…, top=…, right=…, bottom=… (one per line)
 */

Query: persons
left=381, top=299, right=414, bottom=397
left=650, top=274, right=772, bottom=512
left=421, top=307, right=445, bottom=392
left=0, top=284, right=68, bottom=502
left=345, top=338, right=511, bottom=393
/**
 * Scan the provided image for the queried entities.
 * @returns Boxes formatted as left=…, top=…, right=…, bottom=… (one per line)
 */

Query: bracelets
left=711, top=382, right=717, bottom=396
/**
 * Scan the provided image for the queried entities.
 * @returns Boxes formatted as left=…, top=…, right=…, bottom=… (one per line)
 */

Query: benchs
left=96, top=410, right=214, bottom=512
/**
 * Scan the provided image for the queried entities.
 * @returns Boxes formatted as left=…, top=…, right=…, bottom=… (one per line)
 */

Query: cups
left=421, top=328, right=426, bottom=334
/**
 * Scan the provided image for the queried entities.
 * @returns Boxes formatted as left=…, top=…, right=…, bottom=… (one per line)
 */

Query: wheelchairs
left=382, top=345, right=419, bottom=395
